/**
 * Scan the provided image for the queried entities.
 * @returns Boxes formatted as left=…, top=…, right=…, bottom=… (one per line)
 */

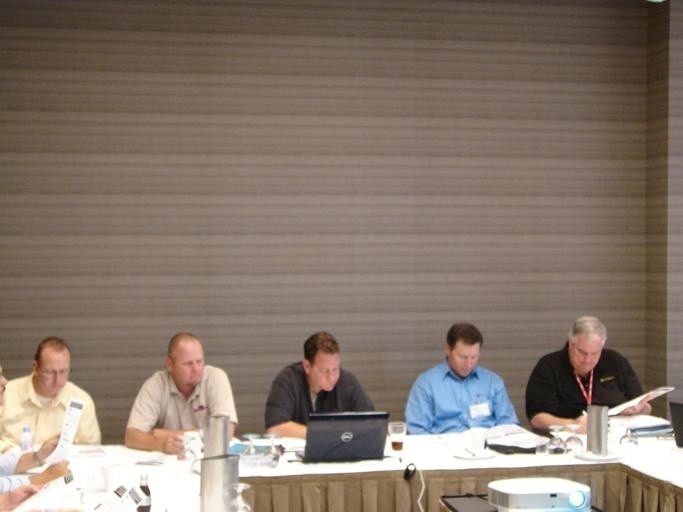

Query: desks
left=0, top=430, right=683, bottom=512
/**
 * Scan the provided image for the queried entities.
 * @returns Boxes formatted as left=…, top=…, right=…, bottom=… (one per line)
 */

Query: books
left=606, top=386, right=675, bottom=437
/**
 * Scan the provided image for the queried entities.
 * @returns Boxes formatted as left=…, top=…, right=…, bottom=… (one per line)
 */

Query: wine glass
left=228, top=480, right=250, bottom=512
left=547, top=422, right=582, bottom=453
left=242, top=432, right=281, bottom=466
left=617, top=422, right=639, bottom=449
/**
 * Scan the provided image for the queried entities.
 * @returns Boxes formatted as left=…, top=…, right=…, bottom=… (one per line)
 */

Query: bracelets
left=33, top=451, right=44, bottom=467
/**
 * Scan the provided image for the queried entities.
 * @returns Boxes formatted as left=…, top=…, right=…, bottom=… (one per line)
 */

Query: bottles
left=136, top=473, right=150, bottom=511
left=19, top=428, right=32, bottom=451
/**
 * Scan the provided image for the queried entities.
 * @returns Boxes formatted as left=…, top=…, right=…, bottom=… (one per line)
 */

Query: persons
left=0, top=364, right=71, bottom=494
left=525, top=316, right=652, bottom=436
left=0, top=336, right=102, bottom=451
left=0, top=484, right=42, bottom=512
left=124, top=332, right=239, bottom=455
left=405, top=323, right=521, bottom=435
left=265, top=332, right=375, bottom=439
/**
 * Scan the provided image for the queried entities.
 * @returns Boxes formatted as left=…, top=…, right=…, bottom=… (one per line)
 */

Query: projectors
left=488, top=477, right=592, bottom=510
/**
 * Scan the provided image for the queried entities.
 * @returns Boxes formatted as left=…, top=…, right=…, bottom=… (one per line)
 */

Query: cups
left=199, top=453, right=241, bottom=512
left=201, top=414, right=230, bottom=456
left=585, top=405, right=608, bottom=456
left=386, top=420, right=405, bottom=449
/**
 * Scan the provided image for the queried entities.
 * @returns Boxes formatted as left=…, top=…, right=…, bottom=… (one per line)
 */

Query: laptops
left=669, top=402, right=683, bottom=446
left=296, top=411, right=389, bottom=463
